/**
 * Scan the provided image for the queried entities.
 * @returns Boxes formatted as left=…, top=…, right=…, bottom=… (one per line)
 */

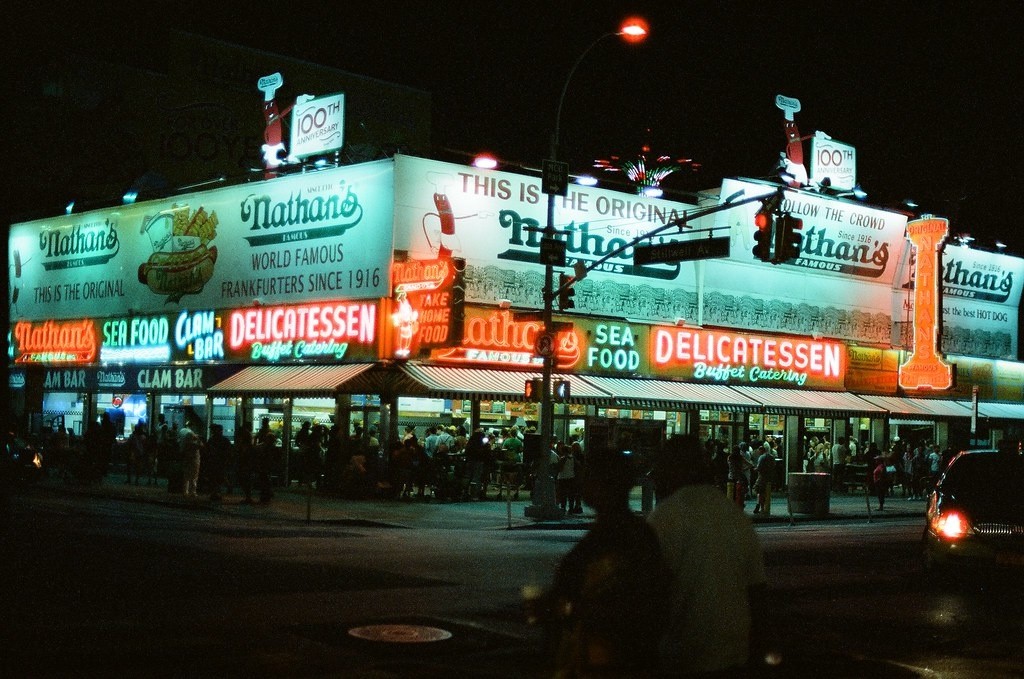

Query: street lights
left=542, top=25, right=645, bottom=440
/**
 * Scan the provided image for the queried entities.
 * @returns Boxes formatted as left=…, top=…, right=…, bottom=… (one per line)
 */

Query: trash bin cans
left=787, top=472, right=831, bottom=514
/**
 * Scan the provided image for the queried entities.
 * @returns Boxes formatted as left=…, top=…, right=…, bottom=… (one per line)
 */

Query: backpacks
left=439, top=437, right=450, bottom=453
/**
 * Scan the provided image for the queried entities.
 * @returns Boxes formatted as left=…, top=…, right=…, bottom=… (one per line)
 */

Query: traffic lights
left=525, top=380, right=542, bottom=402
left=775, top=213, right=802, bottom=262
left=752, top=213, right=772, bottom=261
left=558, top=273, right=575, bottom=308
left=553, top=381, right=569, bottom=403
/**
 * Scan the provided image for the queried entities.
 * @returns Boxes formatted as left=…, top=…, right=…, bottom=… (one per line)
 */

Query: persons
left=542, top=438, right=766, bottom=679
left=56, top=406, right=955, bottom=518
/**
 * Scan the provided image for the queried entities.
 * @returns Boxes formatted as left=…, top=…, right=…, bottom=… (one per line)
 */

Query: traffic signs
left=542, top=159, right=568, bottom=197
left=540, top=237, right=566, bottom=268
left=634, top=236, right=732, bottom=266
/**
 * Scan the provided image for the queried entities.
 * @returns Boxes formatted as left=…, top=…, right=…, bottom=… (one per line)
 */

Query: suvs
left=923, top=450, right=1024, bottom=569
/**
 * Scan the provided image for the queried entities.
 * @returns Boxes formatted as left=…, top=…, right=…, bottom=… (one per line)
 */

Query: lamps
left=978, top=241, right=1007, bottom=251
left=953, top=234, right=976, bottom=246
left=896, top=199, right=918, bottom=209
left=835, top=185, right=867, bottom=198
left=499, top=299, right=513, bottom=309
left=810, top=331, right=824, bottom=340
left=675, top=317, right=687, bottom=327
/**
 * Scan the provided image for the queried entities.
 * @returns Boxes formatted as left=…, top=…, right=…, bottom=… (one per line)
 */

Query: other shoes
left=877, top=507, right=883, bottom=511
left=753, top=510, right=760, bottom=513
left=574, top=508, right=582, bottom=513
left=568, top=509, right=574, bottom=513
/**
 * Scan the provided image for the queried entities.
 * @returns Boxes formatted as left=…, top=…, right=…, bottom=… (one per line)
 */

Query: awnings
left=858, top=394, right=987, bottom=424
left=956, top=401, right=1024, bottom=424
left=577, top=375, right=762, bottom=416
left=728, top=385, right=890, bottom=419
left=397, top=362, right=611, bottom=407
left=206, top=362, right=374, bottom=440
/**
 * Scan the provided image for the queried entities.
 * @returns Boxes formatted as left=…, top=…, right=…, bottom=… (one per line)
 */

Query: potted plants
left=233, top=471, right=270, bottom=504
